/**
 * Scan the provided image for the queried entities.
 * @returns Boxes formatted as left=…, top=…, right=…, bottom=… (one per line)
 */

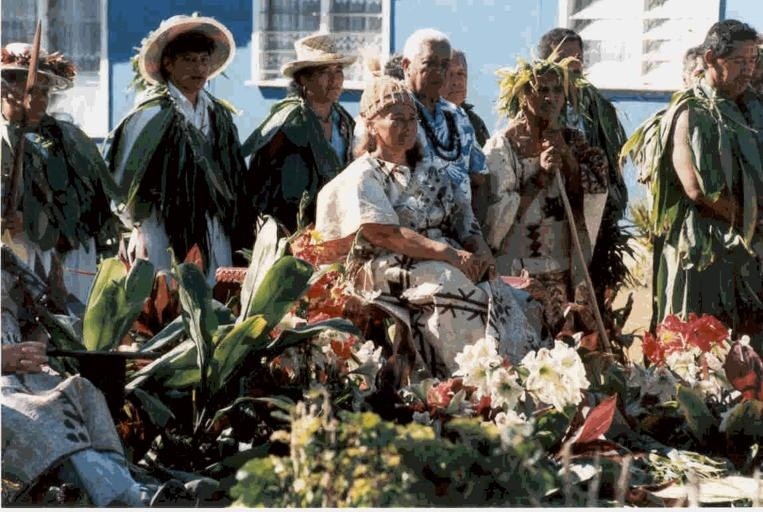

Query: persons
left=0, top=245, right=216, bottom=506
left=242, top=32, right=356, bottom=248
left=0, top=43, right=116, bottom=342
left=618, top=19, right=763, bottom=353
left=100, top=14, right=247, bottom=299
left=317, top=27, right=628, bottom=365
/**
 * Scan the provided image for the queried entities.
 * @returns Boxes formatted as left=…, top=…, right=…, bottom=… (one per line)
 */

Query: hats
left=0, top=42, right=74, bottom=92
left=281, top=34, right=356, bottom=78
left=360, top=78, right=416, bottom=121
left=139, top=15, right=235, bottom=85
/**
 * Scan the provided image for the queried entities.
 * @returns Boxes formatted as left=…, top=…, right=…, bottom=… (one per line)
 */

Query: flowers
left=215, top=310, right=763, bottom=508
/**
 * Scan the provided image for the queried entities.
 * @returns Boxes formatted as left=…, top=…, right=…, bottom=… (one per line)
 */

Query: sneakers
left=149, top=478, right=209, bottom=507
